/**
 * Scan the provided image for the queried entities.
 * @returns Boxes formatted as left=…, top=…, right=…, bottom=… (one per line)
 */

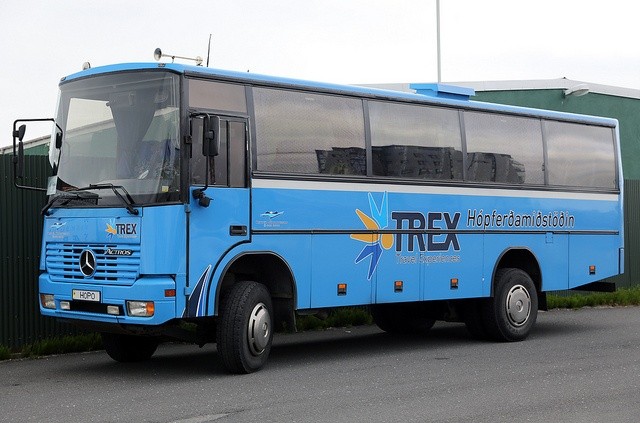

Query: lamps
left=566, top=85, right=589, bottom=97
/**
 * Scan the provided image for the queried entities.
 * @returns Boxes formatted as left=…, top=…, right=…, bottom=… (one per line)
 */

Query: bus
left=13, top=48, right=624, bottom=372
